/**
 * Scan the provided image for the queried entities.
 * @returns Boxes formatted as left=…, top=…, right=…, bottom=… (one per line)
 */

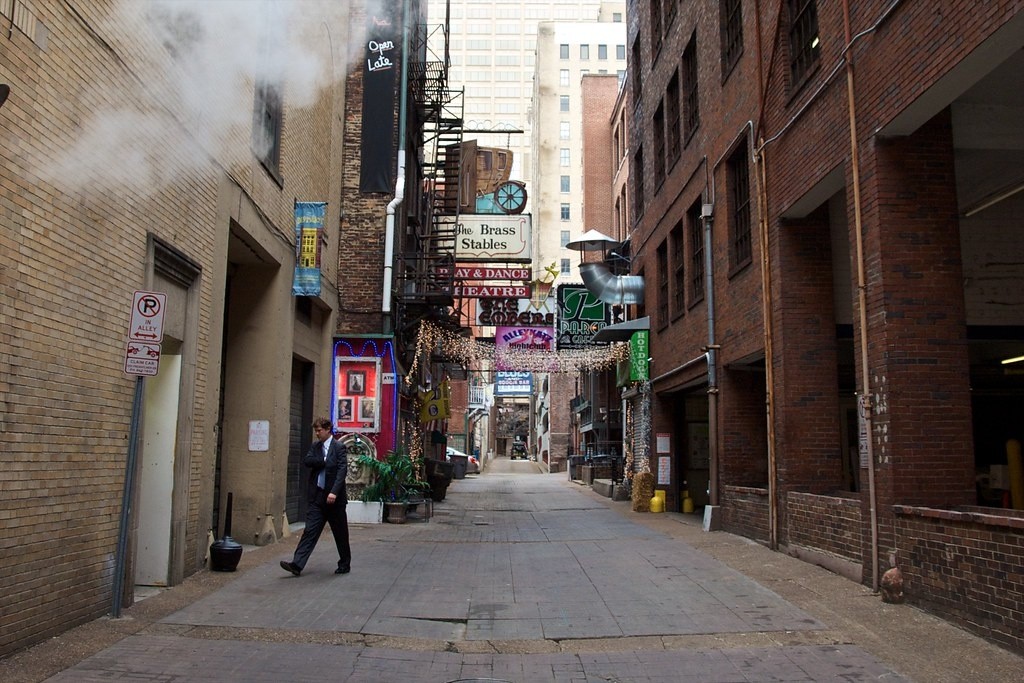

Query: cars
left=446, top=446, right=481, bottom=476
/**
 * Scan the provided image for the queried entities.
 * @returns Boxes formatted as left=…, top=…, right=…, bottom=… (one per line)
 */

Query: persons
left=280, top=418, right=350, bottom=576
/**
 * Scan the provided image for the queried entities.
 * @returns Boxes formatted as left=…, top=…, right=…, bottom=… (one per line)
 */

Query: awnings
left=592, top=315, right=650, bottom=344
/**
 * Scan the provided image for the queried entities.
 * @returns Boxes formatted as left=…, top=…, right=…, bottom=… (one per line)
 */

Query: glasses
left=315, top=429, right=323, bottom=433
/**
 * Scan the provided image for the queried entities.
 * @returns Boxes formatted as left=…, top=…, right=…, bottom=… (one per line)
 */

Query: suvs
left=511, top=442, right=528, bottom=460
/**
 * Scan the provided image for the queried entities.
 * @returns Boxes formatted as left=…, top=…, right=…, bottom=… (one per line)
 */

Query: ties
left=319, top=446, right=325, bottom=488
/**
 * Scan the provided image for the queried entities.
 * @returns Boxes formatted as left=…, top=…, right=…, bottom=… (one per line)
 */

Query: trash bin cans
left=447, top=454, right=468, bottom=481
left=591, top=454, right=614, bottom=486
left=568, top=454, right=585, bottom=481
left=423, top=458, right=455, bottom=503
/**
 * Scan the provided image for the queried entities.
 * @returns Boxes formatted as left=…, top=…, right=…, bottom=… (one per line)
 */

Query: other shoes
left=335, top=567, right=350, bottom=573
left=280, top=561, right=302, bottom=575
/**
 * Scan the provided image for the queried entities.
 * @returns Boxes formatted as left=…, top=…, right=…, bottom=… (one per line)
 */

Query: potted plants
left=345, top=445, right=431, bottom=524
